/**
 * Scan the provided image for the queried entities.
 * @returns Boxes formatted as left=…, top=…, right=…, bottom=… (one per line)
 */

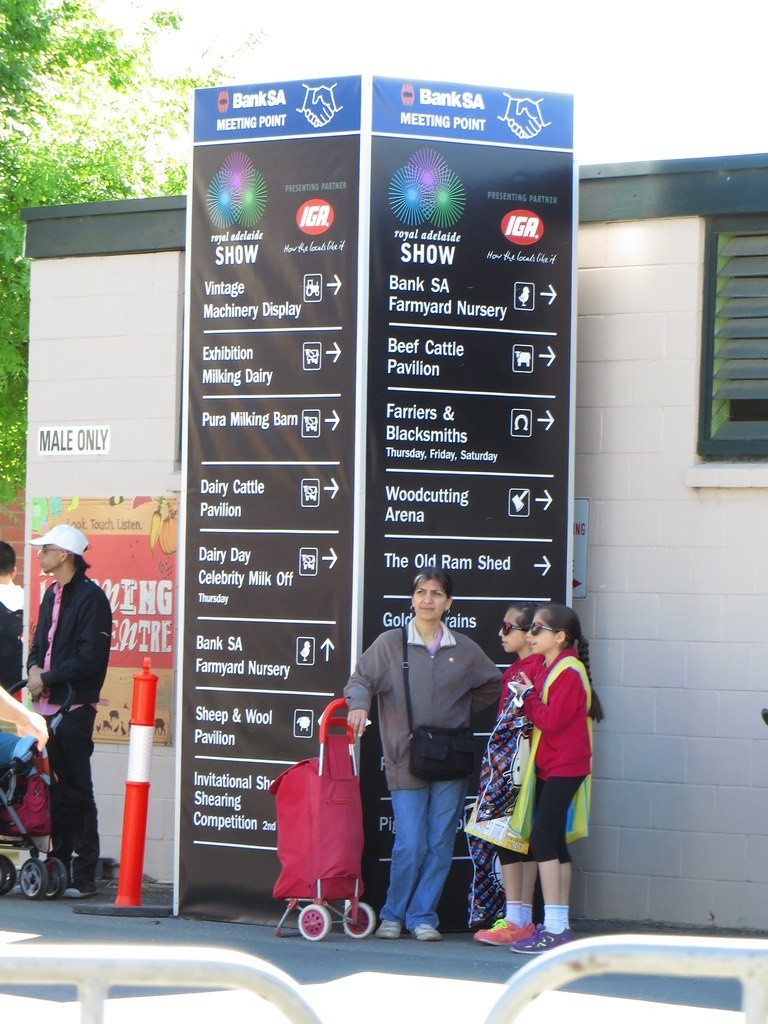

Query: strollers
left=0, top=675, right=76, bottom=899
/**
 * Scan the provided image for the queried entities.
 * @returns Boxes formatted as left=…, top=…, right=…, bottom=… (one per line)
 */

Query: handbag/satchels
left=466, top=830, right=506, bottom=928
left=464, top=797, right=530, bottom=854
left=408, top=724, right=476, bottom=780
left=506, top=750, right=592, bottom=842
left=0, top=751, right=52, bottom=837
left=472, top=681, right=535, bottom=822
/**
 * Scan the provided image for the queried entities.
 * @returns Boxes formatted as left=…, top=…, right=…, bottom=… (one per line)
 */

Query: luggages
left=268, top=699, right=377, bottom=942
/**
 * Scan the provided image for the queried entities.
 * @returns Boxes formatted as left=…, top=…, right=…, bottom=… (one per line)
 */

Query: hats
left=29, top=523, right=88, bottom=556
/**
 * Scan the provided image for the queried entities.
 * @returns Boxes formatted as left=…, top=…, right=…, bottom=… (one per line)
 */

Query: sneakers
left=375, top=919, right=402, bottom=939
left=510, top=923, right=575, bottom=955
left=411, top=924, right=441, bottom=941
left=473, top=918, right=536, bottom=946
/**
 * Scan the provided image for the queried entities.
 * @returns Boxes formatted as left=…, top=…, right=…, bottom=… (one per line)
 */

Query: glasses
left=42, top=546, right=61, bottom=553
left=528, top=622, right=554, bottom=635
left=500, top=621, right=525, bottom=635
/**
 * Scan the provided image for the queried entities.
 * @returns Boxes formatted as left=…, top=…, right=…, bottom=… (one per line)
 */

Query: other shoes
left=63, top=886, right=97, bottom=899
left=15, top=883, right=34, bottom=894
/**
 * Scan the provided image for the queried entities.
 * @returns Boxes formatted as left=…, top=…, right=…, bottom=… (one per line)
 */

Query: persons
left=0, top=523, right=112, bottom=899
left=343, top=567, right=503, bottom=941
left=468, top=602, right=604, bottom=955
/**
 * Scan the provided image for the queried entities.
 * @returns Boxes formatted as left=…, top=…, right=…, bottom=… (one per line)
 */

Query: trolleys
left=275, top=698, right=379, bottom=943
